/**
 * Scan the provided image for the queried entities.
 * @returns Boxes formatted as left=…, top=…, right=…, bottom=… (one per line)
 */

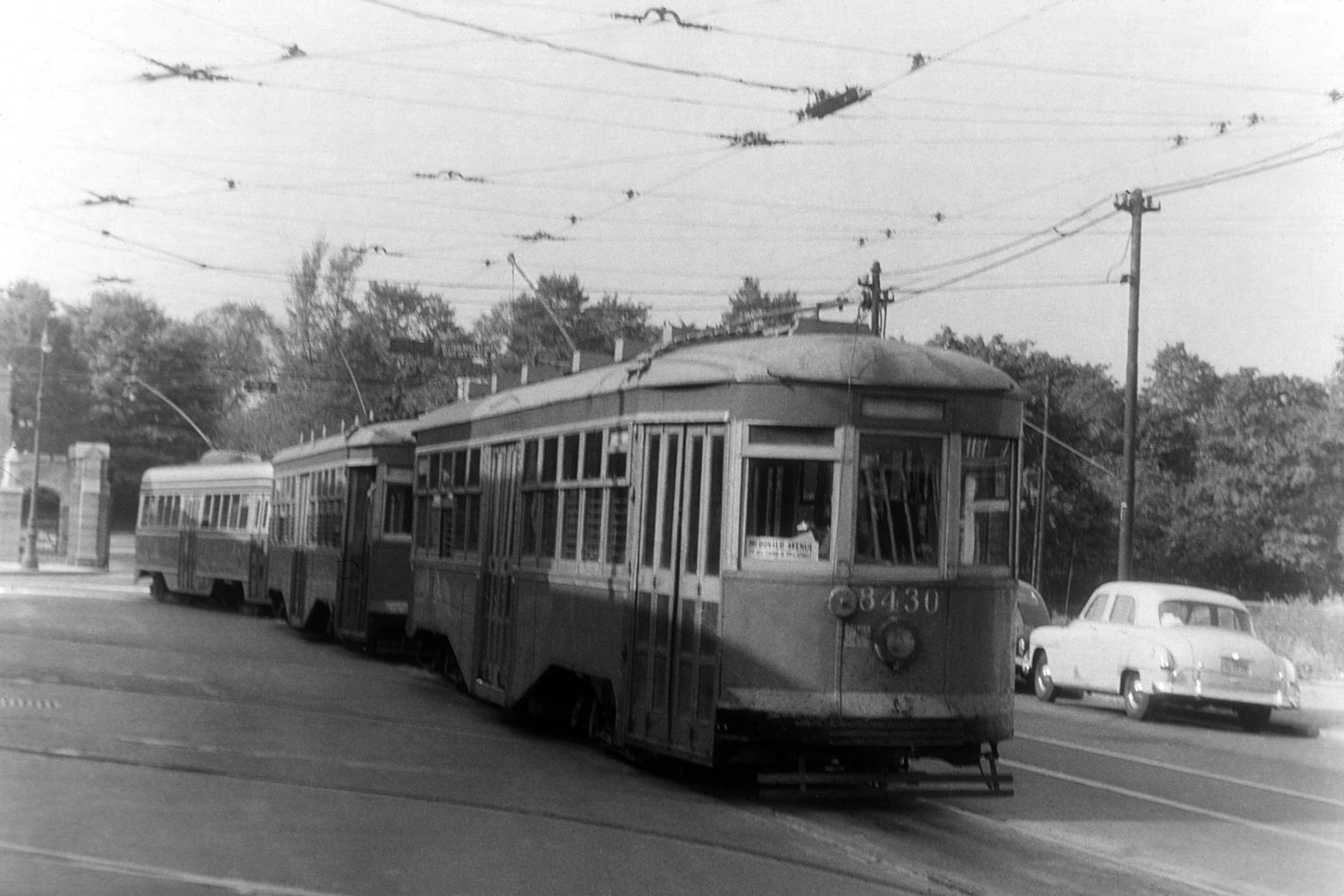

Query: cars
left=1015, top=579, right=1051, bottom=682
left=1026, top=579, right=1302, bottom=732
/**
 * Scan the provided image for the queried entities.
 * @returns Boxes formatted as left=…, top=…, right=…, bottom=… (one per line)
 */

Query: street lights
left=23, top=317, right=55, bottom=570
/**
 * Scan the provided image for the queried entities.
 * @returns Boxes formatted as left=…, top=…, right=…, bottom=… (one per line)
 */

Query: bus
left=410, top=251, right=1030, bottom=802
left=130, top=383, right=274, bottom=616
left=267, top=306, right=417, bottom=654
